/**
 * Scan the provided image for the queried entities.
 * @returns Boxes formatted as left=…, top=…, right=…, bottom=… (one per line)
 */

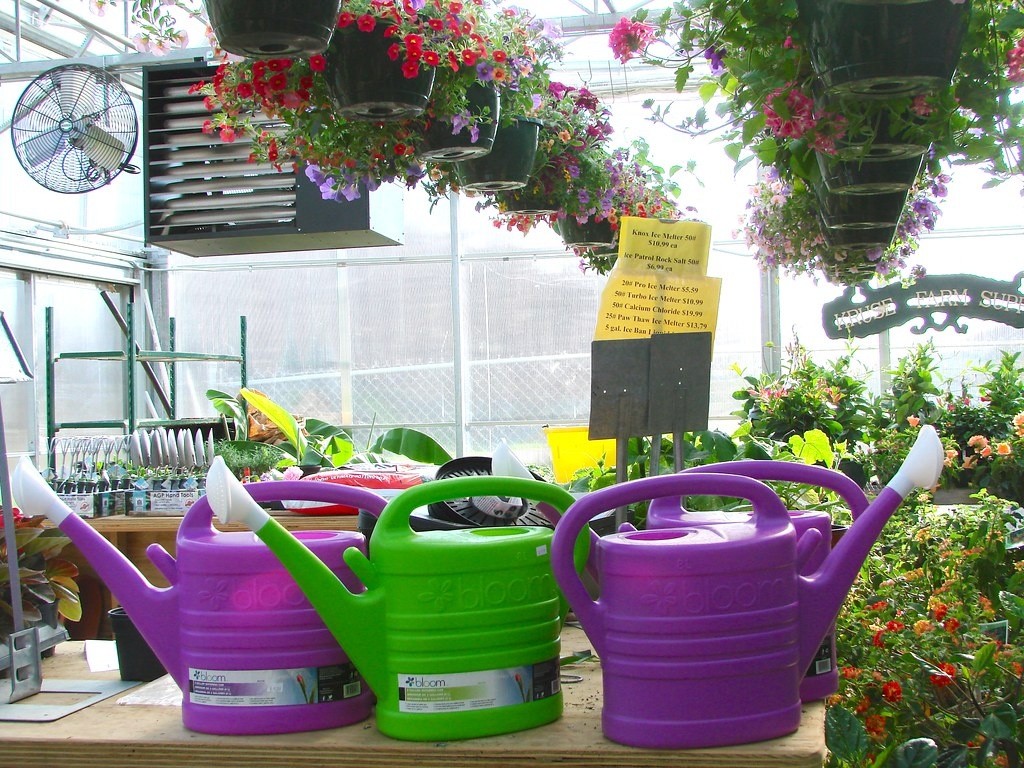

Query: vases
left=414, top=80, right=500, bottom=161
left=815, top=150, right=922, bottom=194
left=498, top=151, right=562, bottom=215
left=953, top=468, right=971, bottom=488
left=203, top=0, right=343, bottom=60
left=815, top=187, right=908, bottom=230
left=557, top=210, right=614, bottom=246
left=592, top=246, right=619, bottom=256
left=837, top=457, right=868, bottom=490
left=821, top=258, right=881, bottom=269
left=325, top=18, right=436, bottom=122
left=817, top=216, right=894, bottom=251
left=823, top=269, right=875, bottom=280
left=810, top=82, right=934, bottom=161
left=798, top=1, right=966, bottom=99
left=453, top=115, right=545, bottom=191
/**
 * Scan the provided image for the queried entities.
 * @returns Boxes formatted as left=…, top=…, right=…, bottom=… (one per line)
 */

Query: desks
left=79, top=510, right=360, bottom=638
left=0, top=643, right=834, bottom=767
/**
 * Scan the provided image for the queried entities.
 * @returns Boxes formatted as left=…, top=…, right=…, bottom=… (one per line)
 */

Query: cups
left=108, top=607, right=168, bottom=681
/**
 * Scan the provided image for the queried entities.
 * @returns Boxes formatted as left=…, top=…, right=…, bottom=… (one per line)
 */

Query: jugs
left=12, top=453, right=390, bottom=734
left=550, top=424, right=945, bottom=749
left=206, top=455, right=588, bottom=743
left=493, top=437, right=871, bottom=703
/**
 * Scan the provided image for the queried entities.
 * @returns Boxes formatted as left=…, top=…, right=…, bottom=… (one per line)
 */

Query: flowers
left=931, top=381, right=1002, bottom=450
left=606, top=0, right=1024, bottom=284
left=188, top=0, right=700, bottom=274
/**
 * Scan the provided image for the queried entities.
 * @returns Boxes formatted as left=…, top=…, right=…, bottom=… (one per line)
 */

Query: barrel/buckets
left=545, top=426, right=616, bottom=483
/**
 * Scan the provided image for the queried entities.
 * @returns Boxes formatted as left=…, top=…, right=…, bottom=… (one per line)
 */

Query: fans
left=10, top=64, right=140, bottom=195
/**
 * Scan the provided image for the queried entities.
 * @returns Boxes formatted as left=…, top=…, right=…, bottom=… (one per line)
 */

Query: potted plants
left=0, top=505, right=82, bottom=673
left=45, top=463, right=210, bottom=516
